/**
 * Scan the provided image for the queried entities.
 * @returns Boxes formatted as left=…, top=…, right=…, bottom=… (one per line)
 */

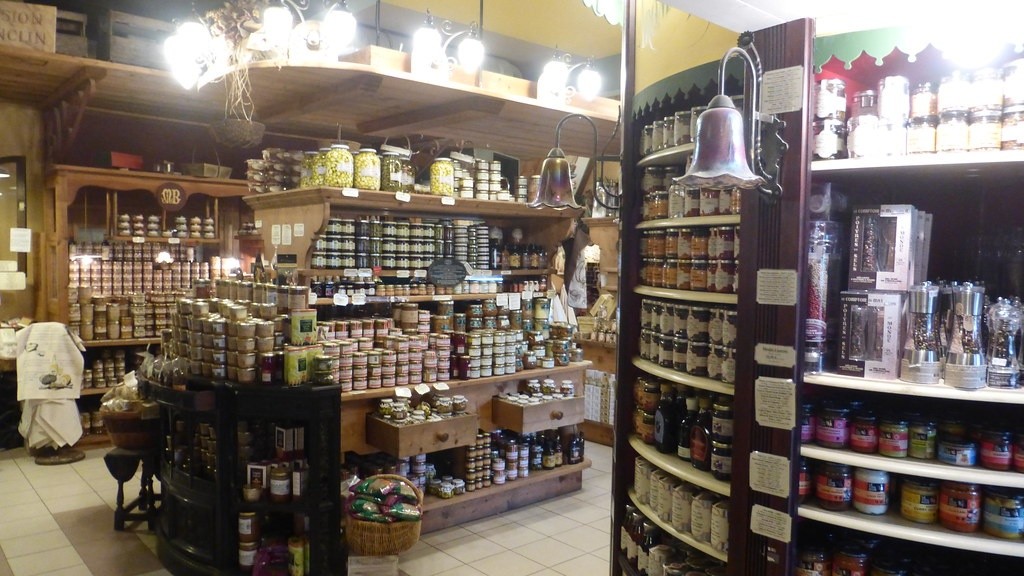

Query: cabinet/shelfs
left=247, top=196, right=589, bottom=539
left=610, top=54, right=751, bottom=574
left=791, top=148, right=1024, bottom=564
left=137, top=373, right=343, bottom=575
left=53, top=167, right=261, bottom=448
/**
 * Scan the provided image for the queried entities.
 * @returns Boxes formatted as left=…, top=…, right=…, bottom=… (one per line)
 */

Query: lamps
left=528, top=113, right=620, bottom=212
left=670, top=46, right=787, bottom=206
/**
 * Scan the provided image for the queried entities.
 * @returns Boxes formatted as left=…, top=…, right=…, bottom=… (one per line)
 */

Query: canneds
left=158, top=293, right=335, bottom=576
left=452, top=159, right=540, bottom=203
left=68, top=242, right=222, bottom=341
left=710, top=401, right=732, bottom=481
left=809, top=56, right=1024, bottom=160
left=794, top=400, right=1024, bottom=576
left=632, top=374, right=662, bottom=445
left=662, top=543, right=728, bottom=576
left=310, top=221, right=584, bottom=497
left=635, top=94, right=748, bottom=387
left=80, top=347, right=126, bottom=436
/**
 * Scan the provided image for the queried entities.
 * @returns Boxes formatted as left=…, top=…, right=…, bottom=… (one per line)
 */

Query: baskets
left=346, top=474, right=423, bottom=553
left=102, top=408, right=161, bottom=449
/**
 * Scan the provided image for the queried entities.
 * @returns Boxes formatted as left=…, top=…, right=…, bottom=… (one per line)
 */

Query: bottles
left=591, top=179, right=616, bottom=218
left=542, top=437, right=563, bottom=469
left=431, top=158, right=453, bottom=195
left=261, top=353, right=273, bottom=382
left=301, top=144, right=415, bottom=192
left=273, top=351, right=284, bottom=381
left=79, top=413, right=107, bottom=435
left=650, top=382, right=712, bottom=471
left=619, top=501, right=661, bottom=576
left=69, top=286, right=132, bottom=341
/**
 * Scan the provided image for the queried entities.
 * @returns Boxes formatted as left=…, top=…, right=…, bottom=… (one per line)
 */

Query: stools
left=104, top=448, right=166, bottom=530
